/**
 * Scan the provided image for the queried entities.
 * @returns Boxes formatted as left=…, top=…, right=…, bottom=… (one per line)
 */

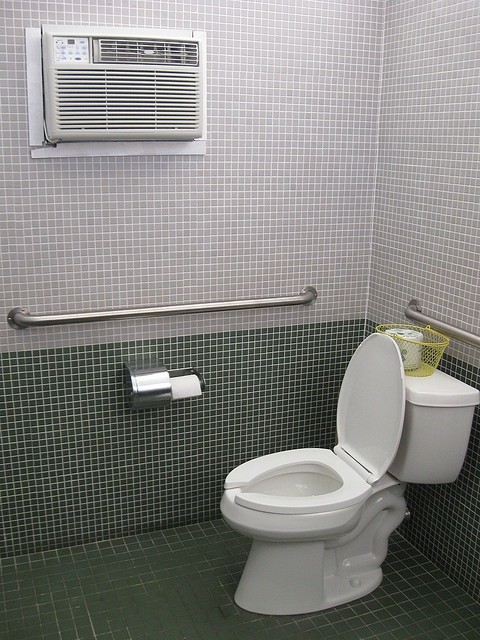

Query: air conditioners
left=39, top=24, right=205, bottom=146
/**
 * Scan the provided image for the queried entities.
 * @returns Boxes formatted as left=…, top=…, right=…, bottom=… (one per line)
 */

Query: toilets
left=220, top=333, right=480, bottom=616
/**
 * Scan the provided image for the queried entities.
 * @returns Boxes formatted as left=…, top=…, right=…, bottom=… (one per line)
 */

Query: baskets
left=375, top=324, right=450, bottom=377
